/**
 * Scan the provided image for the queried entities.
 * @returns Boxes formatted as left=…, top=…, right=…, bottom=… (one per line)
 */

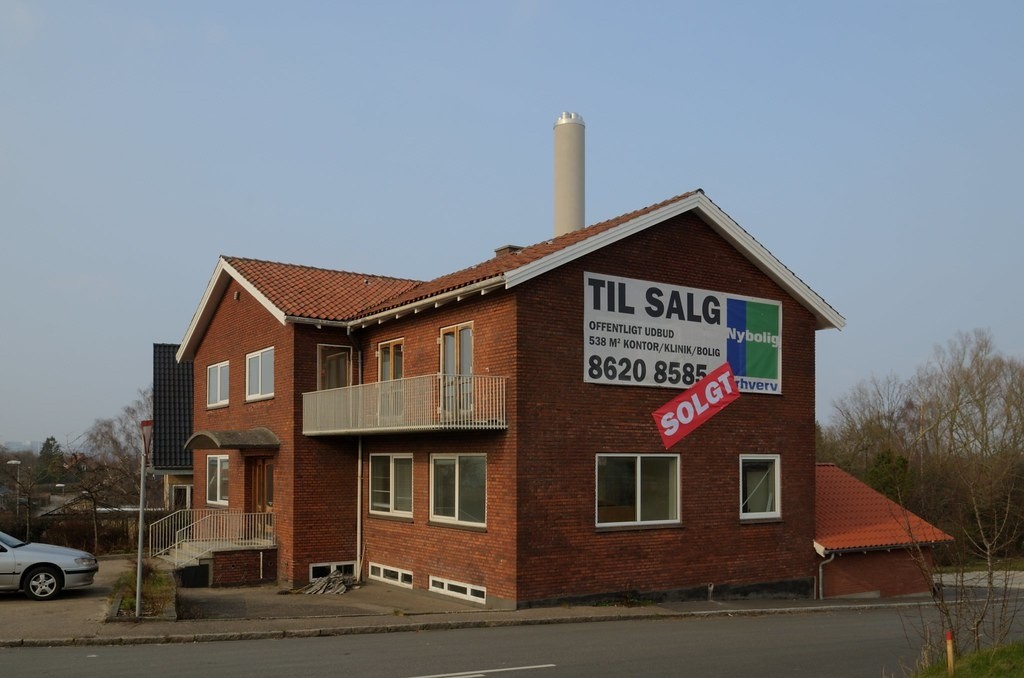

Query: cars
left=0, top=530, right=99, bottom=601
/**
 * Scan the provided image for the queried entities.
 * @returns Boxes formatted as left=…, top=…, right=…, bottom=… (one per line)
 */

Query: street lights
left=6, top=460, right=22, bottom=522
left=56, top=483, right=66, bottom=513
